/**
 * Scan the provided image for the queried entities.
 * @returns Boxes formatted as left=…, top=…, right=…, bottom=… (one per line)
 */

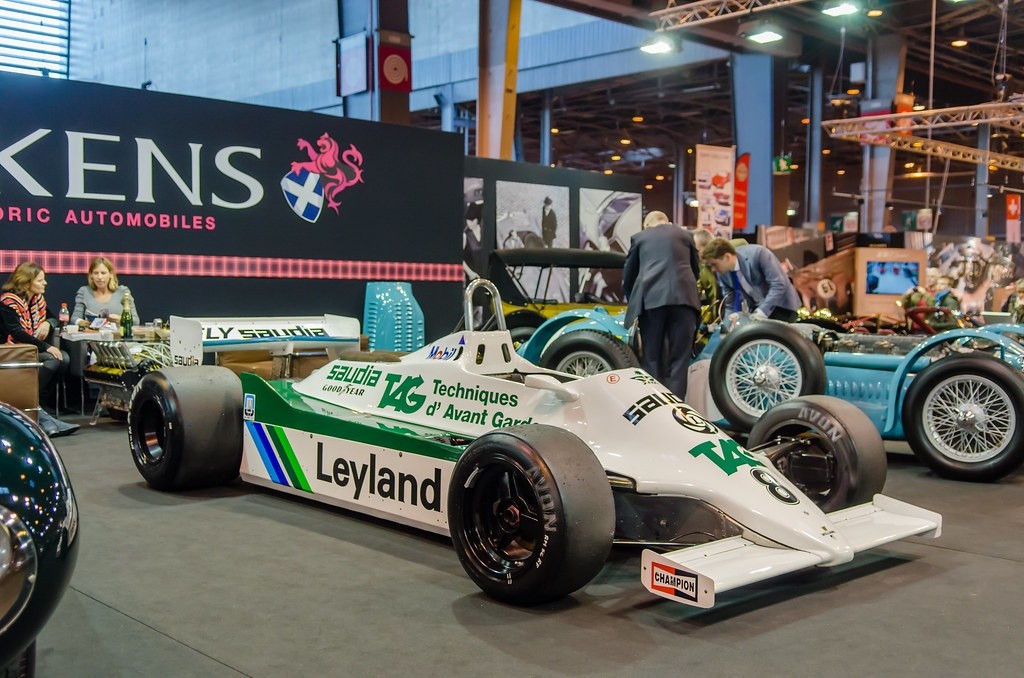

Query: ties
left=730, top=270, right=741, bottom=312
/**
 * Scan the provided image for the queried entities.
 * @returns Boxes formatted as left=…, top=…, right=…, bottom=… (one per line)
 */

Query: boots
left=38, top=404, right=81, bottom=437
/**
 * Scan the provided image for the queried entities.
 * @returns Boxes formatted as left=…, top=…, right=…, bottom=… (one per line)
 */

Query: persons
left=701, top=237, right=801, bottom=322
left=692, top=227, right=749, bottom=317
left=0, top=262, right=70, bottom=417
left=623, top=211, right=699, bottom=402
left=71, top=257, right=140, bottom=329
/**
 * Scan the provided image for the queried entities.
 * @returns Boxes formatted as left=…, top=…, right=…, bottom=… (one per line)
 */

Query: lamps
left=640, top=35, right=683, bottom=57
left=735, top=15, right=786, bottom=45
left=820, top=0, right=885, bottom=18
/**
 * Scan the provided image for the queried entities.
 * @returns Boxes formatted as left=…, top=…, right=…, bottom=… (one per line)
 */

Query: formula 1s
left=515, top=291, right=1024, bottom=481
left=126, top=276, right=944, bottom=612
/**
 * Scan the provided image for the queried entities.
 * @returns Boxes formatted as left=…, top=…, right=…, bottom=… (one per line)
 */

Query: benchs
left=215, top=335, right=411, bottom=380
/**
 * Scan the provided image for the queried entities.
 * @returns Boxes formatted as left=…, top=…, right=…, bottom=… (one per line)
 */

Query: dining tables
left=55, top=325, right=170, bottom=414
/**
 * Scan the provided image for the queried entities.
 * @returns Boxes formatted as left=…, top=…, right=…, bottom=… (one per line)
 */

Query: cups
left=153, top=319, right=163, bottom=329
left=63, top=325, right=78, bottom=334
left=145, top=323, right=155, bottom=339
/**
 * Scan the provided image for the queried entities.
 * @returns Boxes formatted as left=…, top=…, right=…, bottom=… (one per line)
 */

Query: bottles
left=121, top=294, right=134, bottom=339
left=59, top=303, right=69, bottom=336
